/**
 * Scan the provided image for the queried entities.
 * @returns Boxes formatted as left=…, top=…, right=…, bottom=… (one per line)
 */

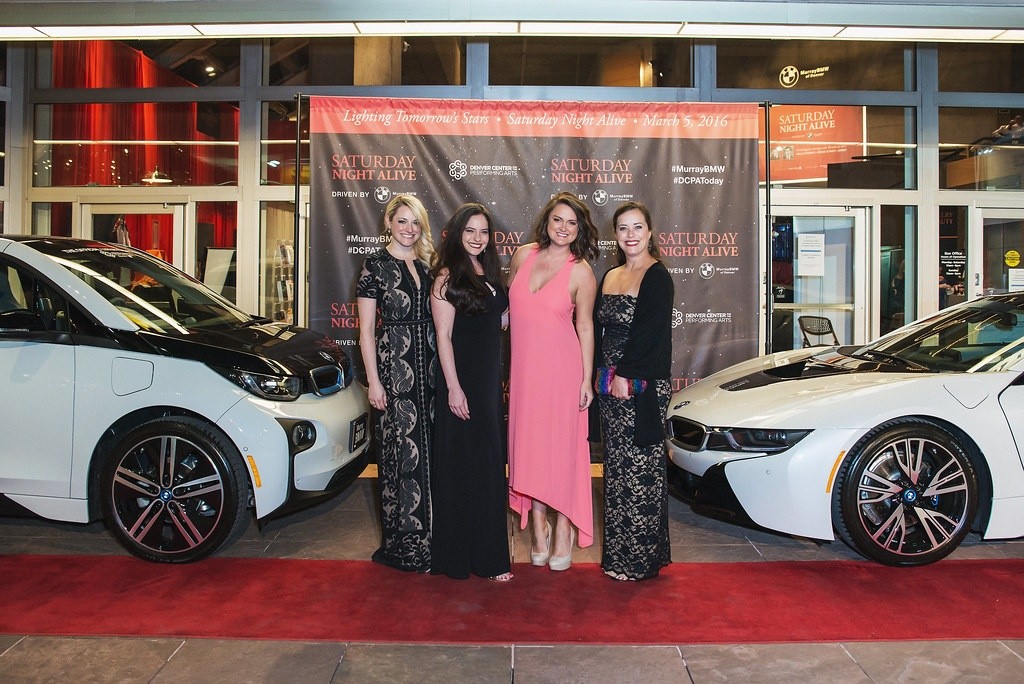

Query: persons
left=592, top=201, right=675, bottom=583
left=504, top=191, right=597, bottom=571
left=939, top=274, right=951, bottom=289
left=428, top=202, right=513, bottom=581
left=356, top=195, right=438, bottom=573
left=885, top=256, right=905, bottom=319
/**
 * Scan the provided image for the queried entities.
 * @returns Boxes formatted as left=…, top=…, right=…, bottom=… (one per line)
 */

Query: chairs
left=0, top=309, right=47, bottom=332
left=798, top=316, right=840, bottom=349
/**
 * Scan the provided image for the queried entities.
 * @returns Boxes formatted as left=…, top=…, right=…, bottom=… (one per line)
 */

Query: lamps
left=142, top=165, right=173, bottom=182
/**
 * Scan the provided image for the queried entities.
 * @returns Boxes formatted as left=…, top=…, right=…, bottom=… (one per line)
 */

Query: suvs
left=0, top=233, right=373, bottom=564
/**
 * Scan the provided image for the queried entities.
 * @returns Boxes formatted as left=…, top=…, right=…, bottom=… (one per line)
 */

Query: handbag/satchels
left=594, top=366, right=648, bottom=396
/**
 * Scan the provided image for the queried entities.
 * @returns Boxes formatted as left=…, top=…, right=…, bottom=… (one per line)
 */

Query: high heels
left=530, top=520, right=552, bottom=566
left=549, top=526, right=576, bottom=571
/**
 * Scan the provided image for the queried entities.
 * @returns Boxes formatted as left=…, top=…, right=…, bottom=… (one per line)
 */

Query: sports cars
left=665, top=290, right=1024, bottom=566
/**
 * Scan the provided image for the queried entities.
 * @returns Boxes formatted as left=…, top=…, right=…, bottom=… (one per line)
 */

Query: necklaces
left=545, top=248, right=570, bottom=273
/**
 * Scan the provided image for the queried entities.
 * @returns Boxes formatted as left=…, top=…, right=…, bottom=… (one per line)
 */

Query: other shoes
left=487, top=572, right=514, bottom=582
left=604, top=570, right=659, bottom=581
